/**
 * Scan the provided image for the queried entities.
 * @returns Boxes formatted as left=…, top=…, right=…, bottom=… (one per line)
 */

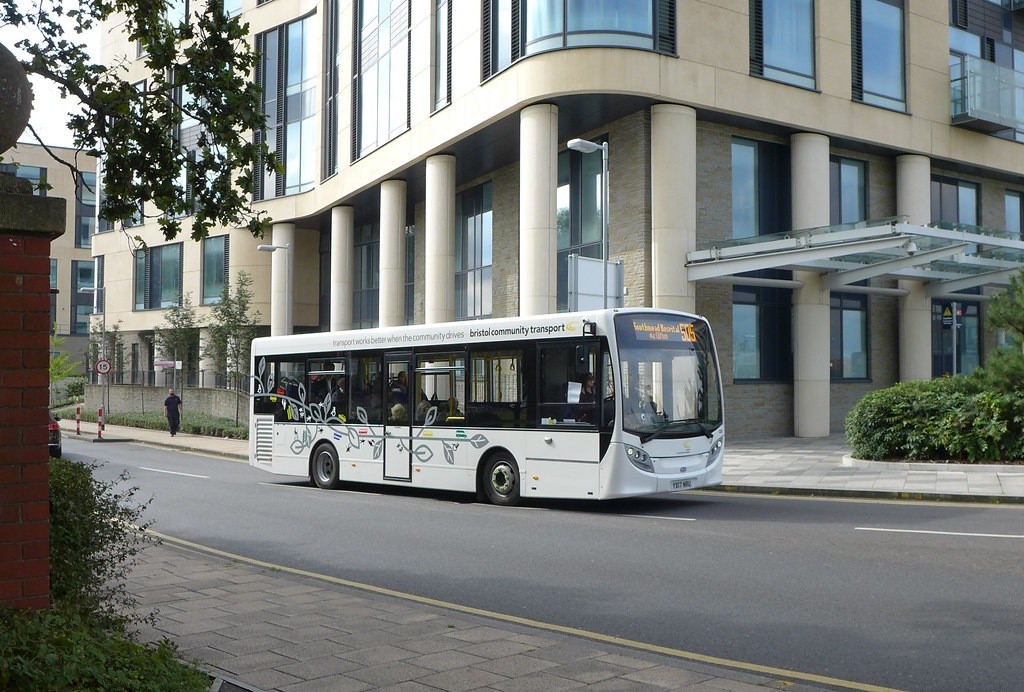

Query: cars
left=48, top=410, right=63, bottom=458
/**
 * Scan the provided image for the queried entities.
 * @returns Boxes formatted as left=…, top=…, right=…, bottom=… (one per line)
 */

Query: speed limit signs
left=95, top=359, right=112, bottom=374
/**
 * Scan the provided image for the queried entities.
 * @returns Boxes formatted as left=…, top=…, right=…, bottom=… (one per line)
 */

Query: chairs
left=437, top=409, right=448, bottom=422
left=421, top=389, right=428, bottom=402
left=286, top=380, right=302, bottom=405
left=367, top=380, right=395, bottom=389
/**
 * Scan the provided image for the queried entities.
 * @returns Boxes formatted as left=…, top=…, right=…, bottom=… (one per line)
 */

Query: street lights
left=566, top=137, right=609, bottom=310
left=79, top=286, right=106, bottom=431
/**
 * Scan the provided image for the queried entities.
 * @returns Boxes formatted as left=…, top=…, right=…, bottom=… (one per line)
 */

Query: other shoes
left=171, top=433, right=174, bottom=437
left=174, top=431, right=176, bottom=435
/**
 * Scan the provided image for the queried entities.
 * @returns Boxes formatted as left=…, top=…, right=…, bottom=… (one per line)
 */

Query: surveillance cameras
left=906, top=243, right=917, bottom=256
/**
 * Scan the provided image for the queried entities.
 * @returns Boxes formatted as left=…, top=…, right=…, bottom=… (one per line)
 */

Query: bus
left=246, top=306, right=726, bottom=507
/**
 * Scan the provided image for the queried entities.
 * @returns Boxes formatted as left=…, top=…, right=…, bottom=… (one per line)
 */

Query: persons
left=314, top=361, right=465, bottom=425
left=164, top=388, right=182, bottom=437
left=577, top=372, right=596, bottom=420
